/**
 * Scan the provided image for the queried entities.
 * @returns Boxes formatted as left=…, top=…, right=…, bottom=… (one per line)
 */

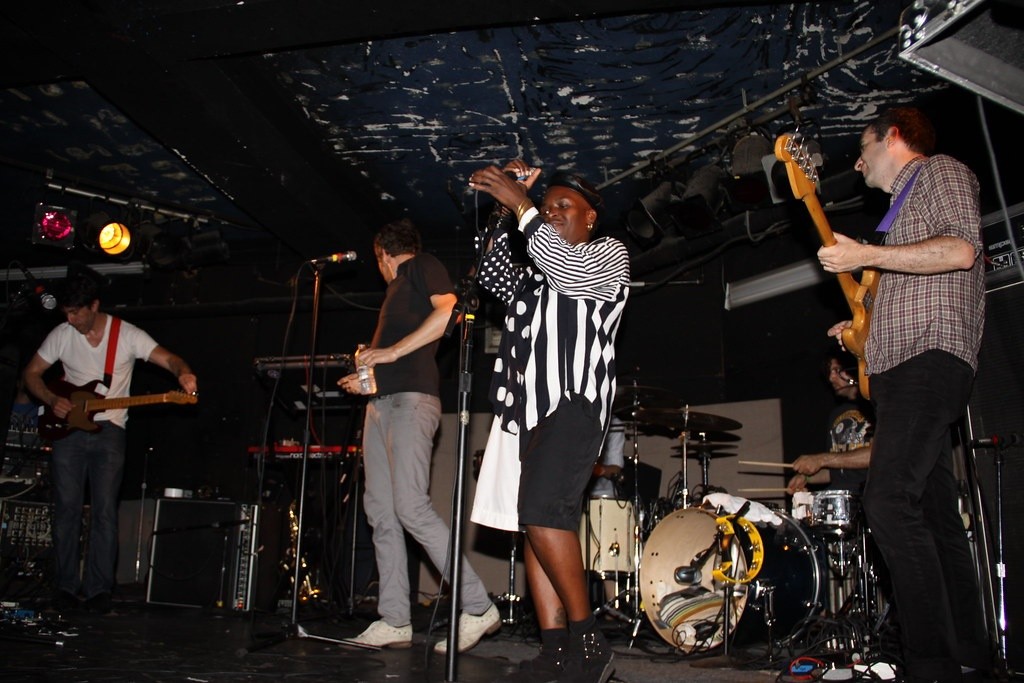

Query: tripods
left=245, top=264, right=380, bottom=653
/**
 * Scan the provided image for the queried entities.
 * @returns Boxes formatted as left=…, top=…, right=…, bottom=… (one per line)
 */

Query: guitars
left=37, top=379, right=195, bottom=437
left=776, top=131, right=883, bottom=399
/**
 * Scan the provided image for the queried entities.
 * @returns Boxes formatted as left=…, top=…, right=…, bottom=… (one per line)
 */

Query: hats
left=546, top=172, right=605, bottom=221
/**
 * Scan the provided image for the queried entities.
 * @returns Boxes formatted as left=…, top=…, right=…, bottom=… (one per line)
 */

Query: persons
left=23, top=275, right=199, bottom=610
left=787, top=348, right=877, bottom=496
left=338, top=220, right=503, bottom=652
left=817, top=105, right=1000, bottom=683
left=469, top=158, right=630, bottom=683
left=1, top=371, right=48, bottom=446
left=583, top=415, right=626, bottom=638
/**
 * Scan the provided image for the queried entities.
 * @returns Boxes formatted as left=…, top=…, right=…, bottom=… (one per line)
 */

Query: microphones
left=482, top=170, right=518, bottom=187
left=311, top=251, right=357, bottom=264
left=16, top=262, right=57, bottom=308
left=968, top=432, right=1020, bottom=446
left=676, top=565, right=702, bottom=585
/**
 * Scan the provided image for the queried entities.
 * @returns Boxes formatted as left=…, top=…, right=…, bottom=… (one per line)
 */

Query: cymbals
left=632, top=409, right=743, bottom=427
left=670, top=441, right=741, bottom=452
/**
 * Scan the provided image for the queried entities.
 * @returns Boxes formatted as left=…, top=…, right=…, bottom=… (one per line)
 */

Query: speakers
left=955, top=263, right=1023, bottom=676
left=145, top=498, right=240, bottom=610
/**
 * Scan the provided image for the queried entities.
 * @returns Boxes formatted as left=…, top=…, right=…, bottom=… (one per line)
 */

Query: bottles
left=355, top=344, right=377, bottom=395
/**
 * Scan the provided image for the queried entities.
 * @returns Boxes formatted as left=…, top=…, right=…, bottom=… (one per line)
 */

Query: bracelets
left=515, top=197, right=532, bottom=223
left=806, top=474, right=811, bottom=485
left=600, top=466, right=605, bottom=476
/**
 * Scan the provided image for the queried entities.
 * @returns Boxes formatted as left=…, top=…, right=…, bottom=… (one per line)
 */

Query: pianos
left=248, top=444, right=362, bottom=461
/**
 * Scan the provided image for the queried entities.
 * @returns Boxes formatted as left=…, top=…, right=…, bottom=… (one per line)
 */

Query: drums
left=581, top=496, right=638, bottom=576
left=638, top=505, right=822, bottom=654
left=810, top=489, right=865, bottom=541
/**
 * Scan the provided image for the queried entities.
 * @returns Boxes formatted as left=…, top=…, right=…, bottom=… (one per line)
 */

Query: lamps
left=32, top=186, right=231, bottom=263
left=626, top=124, right=772, bottom=239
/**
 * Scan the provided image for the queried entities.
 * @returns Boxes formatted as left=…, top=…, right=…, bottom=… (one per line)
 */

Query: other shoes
left=90, top=592, right=111, bottom=614
left=435, top=602, right=502, bottom=655
left=557, top=629, right=615, bottom=683
left=339, top=620, right=413, bottom=650
left=51, top=591, right=76, bottom=606
left=501, top=648, right=565, bottom=683
